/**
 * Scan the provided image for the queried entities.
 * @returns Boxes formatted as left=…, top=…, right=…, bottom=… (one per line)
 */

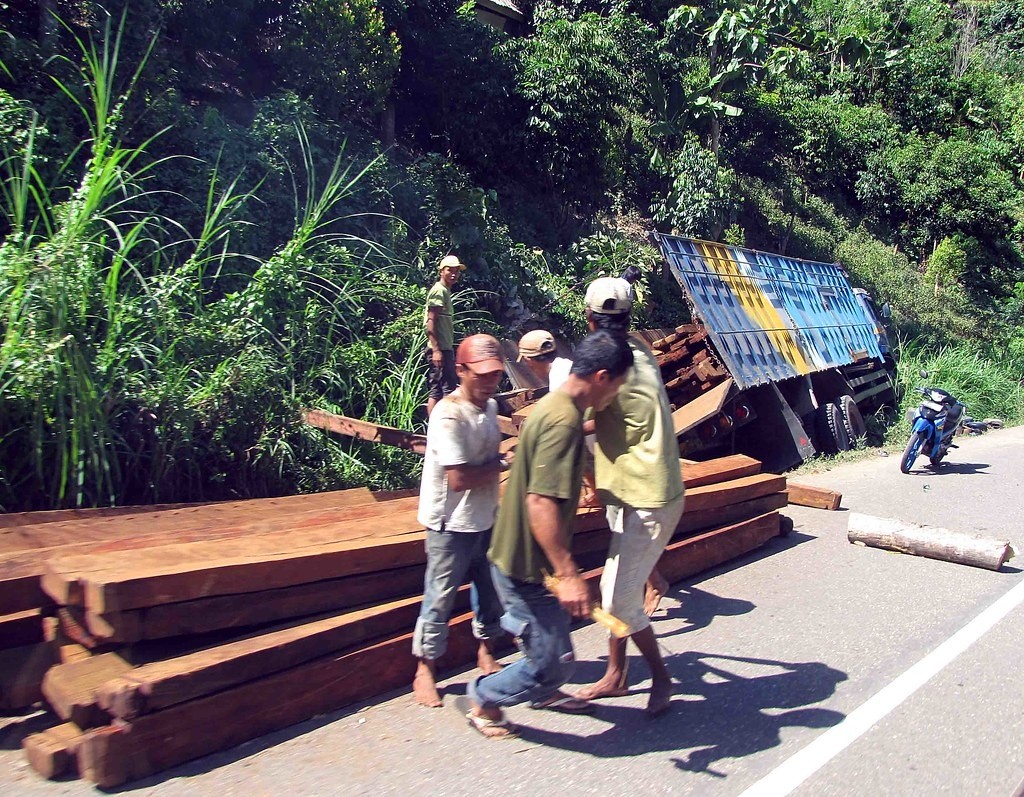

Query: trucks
left=496, top=232, right=900, bottom=470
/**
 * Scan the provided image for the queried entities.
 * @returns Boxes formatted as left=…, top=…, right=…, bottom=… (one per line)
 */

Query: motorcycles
left=899, top=368, right=969, bottom=473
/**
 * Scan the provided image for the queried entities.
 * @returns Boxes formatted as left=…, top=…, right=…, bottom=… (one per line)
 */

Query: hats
left=584, top=277, right=635, bottom=313
left=438, top=255, right=465, bottom=269
left=457, top=334, right=504, bottom=374
left=516, top=329, right=556, bottom=362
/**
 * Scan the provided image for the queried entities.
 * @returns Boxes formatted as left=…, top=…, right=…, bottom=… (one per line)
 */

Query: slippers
left=531, top=690, right=590, bottom=715
left=466, top=707, right=521, bottom=739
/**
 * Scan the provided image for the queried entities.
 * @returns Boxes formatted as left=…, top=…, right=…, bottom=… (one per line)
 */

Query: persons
left=404, top=334, right=524, bottom=707
left=510, top=326, right=671, bottom=620
left=463, top=330, right=636, bottom=742
left=568, top=273, right=692, bottom=721
left=422, top=253, right=466, bottom=420
